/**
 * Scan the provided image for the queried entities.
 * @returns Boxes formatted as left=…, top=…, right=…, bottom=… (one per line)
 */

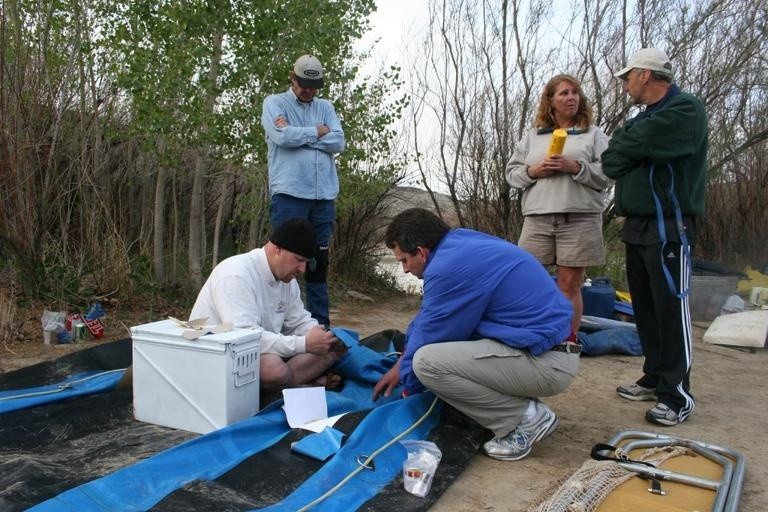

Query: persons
left=504, top=73, right=617, bottom=337
left=600, top=48, right=710, bottom=426
left=371, top=207, right=584, bottom=462
left=188, top=218, right=349, bottom=393
left=261, top=54, right=346, bottom=331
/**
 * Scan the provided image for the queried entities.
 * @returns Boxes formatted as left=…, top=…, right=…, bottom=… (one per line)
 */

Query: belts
left=552, top=344, right=580, bottom=354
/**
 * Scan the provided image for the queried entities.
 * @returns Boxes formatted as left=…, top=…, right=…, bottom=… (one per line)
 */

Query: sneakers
left=616, top=384, right=688, bottom=426
left=482, top=400, right=559, bottom=461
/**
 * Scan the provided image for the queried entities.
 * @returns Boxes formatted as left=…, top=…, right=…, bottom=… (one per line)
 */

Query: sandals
left=310, top=369, right=343, bottom=390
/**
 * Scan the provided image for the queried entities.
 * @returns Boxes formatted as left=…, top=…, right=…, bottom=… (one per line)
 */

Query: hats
left=294, top=54, right=324, bottom=88
left=269, top=218, right=316, bottom=258
left=613, top=48, right=672, bottom=77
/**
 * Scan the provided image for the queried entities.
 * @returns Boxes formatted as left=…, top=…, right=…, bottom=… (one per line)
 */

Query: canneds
left=74, top=322, right=87, bottom=343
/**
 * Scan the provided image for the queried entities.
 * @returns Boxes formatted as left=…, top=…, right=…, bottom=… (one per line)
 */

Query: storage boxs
left=129, top=319, right=262, bottom=435
